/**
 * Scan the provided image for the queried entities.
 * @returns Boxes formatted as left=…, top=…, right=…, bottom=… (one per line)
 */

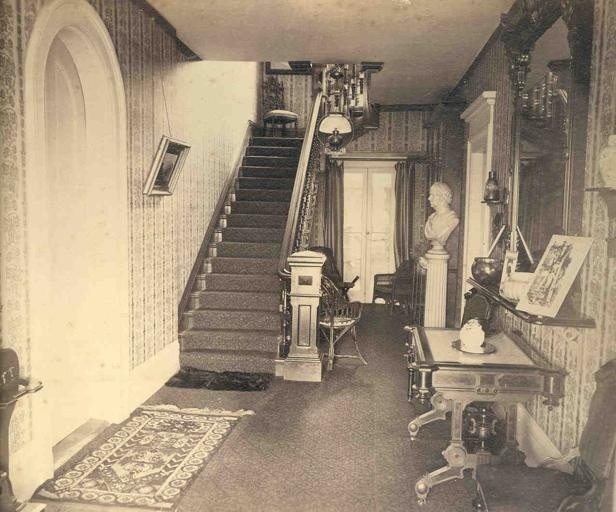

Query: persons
left=424, top=181, right=460, bottom=246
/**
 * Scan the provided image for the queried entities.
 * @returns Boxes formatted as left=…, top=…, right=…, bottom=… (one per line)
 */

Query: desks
left=405, top=325, right=566, bottom=505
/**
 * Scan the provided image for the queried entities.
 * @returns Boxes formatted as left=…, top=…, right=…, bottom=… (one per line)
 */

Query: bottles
left=484, top=170, right=499, bottom=201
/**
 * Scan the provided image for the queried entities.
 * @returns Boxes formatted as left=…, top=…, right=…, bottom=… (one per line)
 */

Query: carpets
left=29, top=405, right=255, bottom=511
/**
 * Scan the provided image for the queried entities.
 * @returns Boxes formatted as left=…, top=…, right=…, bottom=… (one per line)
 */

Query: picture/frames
left=141, top=134, right=192, bottom=197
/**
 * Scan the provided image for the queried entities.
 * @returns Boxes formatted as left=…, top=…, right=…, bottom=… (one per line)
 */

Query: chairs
left=372, top=261, right=414, bottom=316
left=309, top=246, right=354, bottom=301
left=262, top=76, right=298, bottom=136
left=475, top=359, right=614, bottom=512
left=404, top=289, right=495, bottom=401
left=318, top=274, right=367, bottom=370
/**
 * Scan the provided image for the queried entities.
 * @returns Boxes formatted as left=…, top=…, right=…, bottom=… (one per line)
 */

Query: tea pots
left=460, top=319, right=485, bottom=346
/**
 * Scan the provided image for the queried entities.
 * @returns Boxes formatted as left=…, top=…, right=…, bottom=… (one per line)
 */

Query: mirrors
left=500, top=0, right=593, bottom=300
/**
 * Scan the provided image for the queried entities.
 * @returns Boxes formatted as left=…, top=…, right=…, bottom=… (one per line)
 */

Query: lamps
left=318, top=114, right=352, bottom=150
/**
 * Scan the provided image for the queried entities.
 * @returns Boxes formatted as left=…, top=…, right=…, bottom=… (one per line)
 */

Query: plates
left=451, top=339, right=496, bottom=355
